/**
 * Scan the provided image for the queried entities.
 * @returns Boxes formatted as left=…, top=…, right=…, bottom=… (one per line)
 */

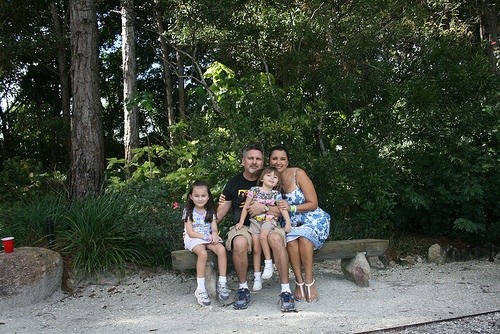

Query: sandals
left=305, top=278, right=319, bottom=302
left=294, top=281, right=307, bottom=303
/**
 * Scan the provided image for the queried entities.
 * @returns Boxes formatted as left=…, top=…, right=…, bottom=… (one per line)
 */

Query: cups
left=1, top=237, right=15, bottom=252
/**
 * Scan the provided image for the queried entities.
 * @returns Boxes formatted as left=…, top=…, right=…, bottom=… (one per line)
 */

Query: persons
left=269, top=146, right=330, bottom=304
left=183, top=148, right=297, bottom=312
left=235, top=165, right=292, bottom=292
left=182, top=180, right=231, bottom=306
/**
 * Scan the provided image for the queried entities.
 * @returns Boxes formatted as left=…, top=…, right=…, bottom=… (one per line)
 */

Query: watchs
left=264, top=204, right=270, bottom=214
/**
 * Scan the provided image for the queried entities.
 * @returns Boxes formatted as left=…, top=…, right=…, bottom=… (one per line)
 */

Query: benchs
left=172, top=238, right=388, bottom=300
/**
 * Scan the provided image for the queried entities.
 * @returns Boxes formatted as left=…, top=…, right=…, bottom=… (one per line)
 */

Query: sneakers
left=252, top=277, right=263, bottom=291
left=216, top=280, right=231, bottom=300
left=233, top=288, right=250, bottom=309
left=278, top=291, right=295, bottom=311
left=195, top=288, right=211, bottom=306
left=262, top=265, right=275, bottom=280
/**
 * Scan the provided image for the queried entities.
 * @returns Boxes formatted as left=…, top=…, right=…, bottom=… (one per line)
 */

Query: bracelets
left=290, top=205, right=296, bottom=216
